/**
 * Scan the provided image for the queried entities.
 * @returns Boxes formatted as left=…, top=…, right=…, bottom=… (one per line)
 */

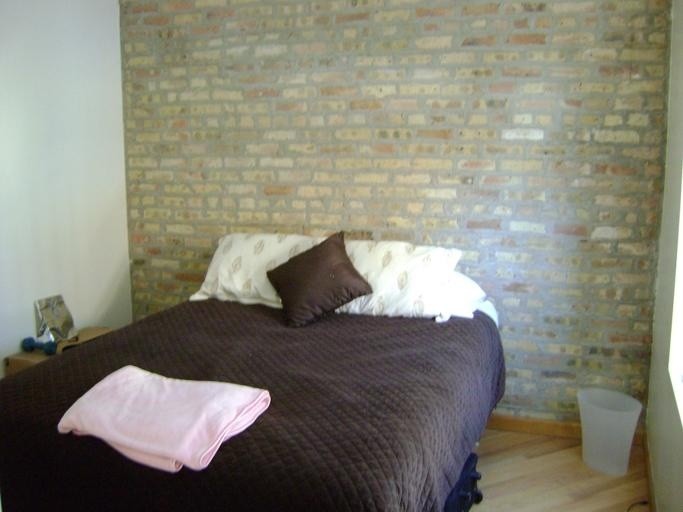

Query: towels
left=56, top=361, right=273, bottom=475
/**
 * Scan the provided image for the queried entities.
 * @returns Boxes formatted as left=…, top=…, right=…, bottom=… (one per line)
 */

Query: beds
left=0, top=298, right=503, bottom=509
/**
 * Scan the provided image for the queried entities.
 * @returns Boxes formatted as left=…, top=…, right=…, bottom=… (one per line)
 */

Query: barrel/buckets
left=577, top=388, right=644, bottom=477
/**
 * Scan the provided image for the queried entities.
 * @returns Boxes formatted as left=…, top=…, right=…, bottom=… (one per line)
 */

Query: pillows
left=267, top=229, right=374, bottom=325
left=185, top=234, right=326, bottom=309
left=335, top=238, right=467, bottom=320
